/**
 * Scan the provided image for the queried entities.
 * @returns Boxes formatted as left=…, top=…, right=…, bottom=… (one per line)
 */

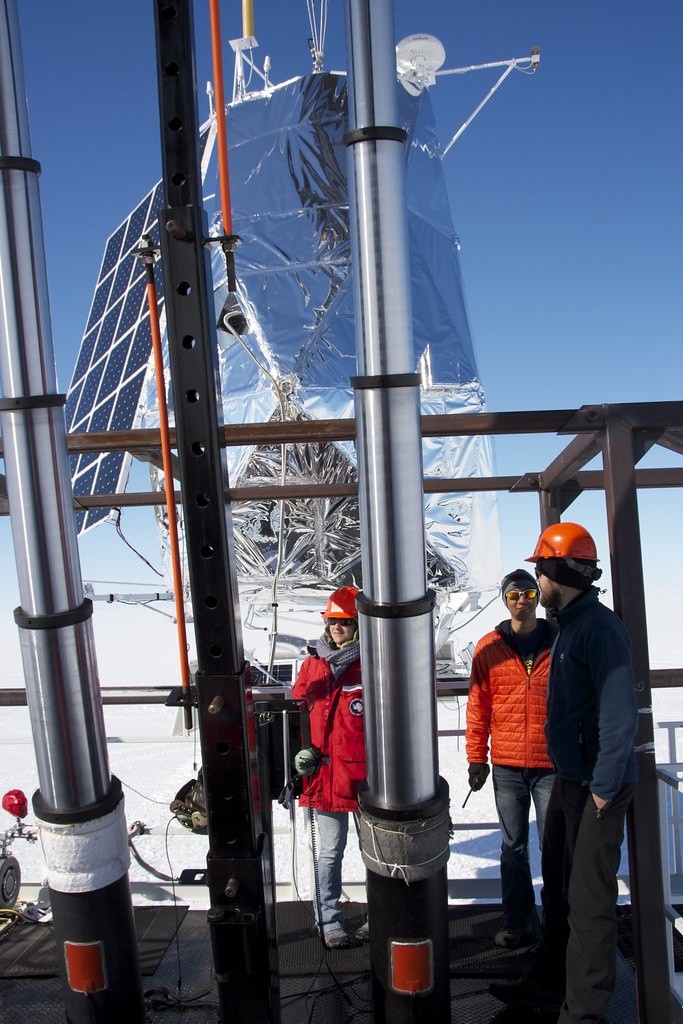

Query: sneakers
left=318, top=925, right=348, bottom=948
left=356, top=920, right=370, bottom=942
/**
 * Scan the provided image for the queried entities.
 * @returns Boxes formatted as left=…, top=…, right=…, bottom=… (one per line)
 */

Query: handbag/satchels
left=295, top=745, right=321, bottom=776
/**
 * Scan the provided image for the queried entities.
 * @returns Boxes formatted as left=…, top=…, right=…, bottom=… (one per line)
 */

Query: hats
left=501, top=569, right=540, bottom=607
left=536, top=557, right=596, bottom=591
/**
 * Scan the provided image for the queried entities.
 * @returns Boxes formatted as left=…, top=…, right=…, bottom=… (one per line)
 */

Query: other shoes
left=495, top=926, right=528, bottom=947
left=488, top=977, right=567, bottom=1015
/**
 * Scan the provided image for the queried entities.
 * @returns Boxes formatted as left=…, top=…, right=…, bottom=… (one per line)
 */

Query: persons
left=292, top=586, right=370, bottom=946
left=466, top=570, right=555, bottom=948
left=488, top=522, right=640, bottom=1024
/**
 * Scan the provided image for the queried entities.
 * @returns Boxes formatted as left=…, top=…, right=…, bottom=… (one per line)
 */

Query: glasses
left=506, top=588, right=539, bottom=600
left=327, top=618, right=356, bottom=626
left=534, top=566, right=542, bottom=579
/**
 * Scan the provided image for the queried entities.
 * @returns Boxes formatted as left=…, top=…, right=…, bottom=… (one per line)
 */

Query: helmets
left=322, top=586, right=360, bottom=618
left=525, top=522, right=600, bottom=563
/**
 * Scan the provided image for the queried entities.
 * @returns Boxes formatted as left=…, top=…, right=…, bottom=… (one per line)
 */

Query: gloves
left=468, top=762, right=490, bottom=791
